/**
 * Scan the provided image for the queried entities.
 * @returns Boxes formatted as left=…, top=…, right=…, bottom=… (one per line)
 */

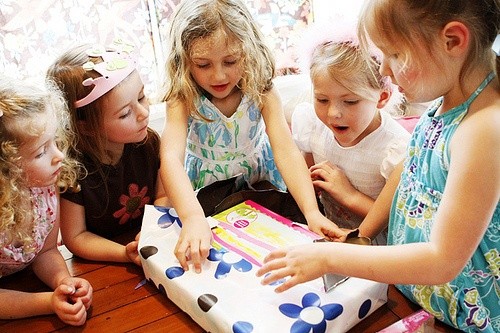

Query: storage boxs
left=138, top=201, right=388, bottom=333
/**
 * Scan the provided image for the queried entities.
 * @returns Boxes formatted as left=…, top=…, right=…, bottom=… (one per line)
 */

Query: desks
left=0, top=244, right=422, bottom=333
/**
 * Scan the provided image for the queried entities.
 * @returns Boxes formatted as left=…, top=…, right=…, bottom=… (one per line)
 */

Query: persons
left=0, top=78, right=93, bottom=327
left=46, top=43, right=173, bottom=267
left=158, top=1, right=348, bottom=273
left=255, top=1, right=500, bottom=333
left=291, top=38, right=412, bottom=246
